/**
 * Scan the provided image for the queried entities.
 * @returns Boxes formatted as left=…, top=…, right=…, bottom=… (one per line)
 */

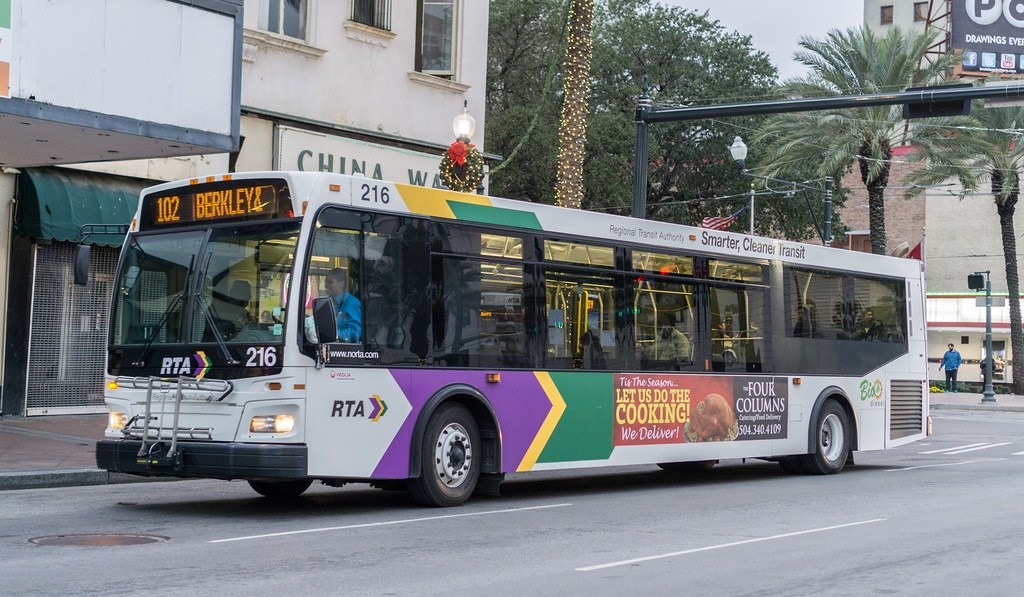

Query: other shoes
left=953, top=390, right=958, bottom=393
left=945, top=389, right=951, bottom=393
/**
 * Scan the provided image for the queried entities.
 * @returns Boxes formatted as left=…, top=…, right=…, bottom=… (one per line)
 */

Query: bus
left=69, top=168, right=934, bottom=510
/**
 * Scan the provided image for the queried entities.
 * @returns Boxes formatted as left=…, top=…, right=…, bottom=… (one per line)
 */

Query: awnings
left=12, top=169, right=157, bottom=249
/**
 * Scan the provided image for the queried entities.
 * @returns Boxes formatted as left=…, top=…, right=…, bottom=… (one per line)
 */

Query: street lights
left=453, top=99, right=486, bottom=196
left=730, top=134, right=834, bottom=248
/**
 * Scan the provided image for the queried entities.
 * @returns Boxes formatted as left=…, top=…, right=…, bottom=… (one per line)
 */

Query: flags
left=701, top=208, right=744, bottom=230
left=906, top=242, right=924, bottom=271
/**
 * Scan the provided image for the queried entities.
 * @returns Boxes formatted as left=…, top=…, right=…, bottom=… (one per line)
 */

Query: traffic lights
left=967, top=275, right=984, bottom=290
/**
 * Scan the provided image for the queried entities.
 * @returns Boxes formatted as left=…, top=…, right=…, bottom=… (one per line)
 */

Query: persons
left=938, top=343, right=962, bottom=393
left=649, top=302, right=881, bottom=358
left=980, top=355, right=996, bottom=393
left=202, top=266, right=362, bottom=344
